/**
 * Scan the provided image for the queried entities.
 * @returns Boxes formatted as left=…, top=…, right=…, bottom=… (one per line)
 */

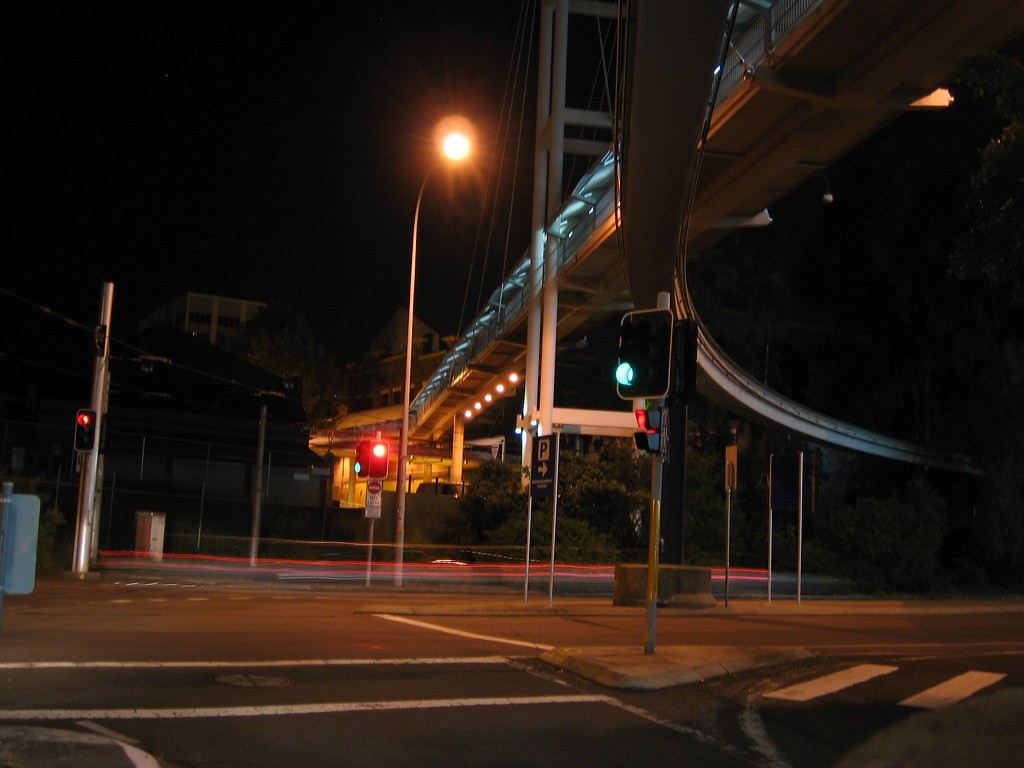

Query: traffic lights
left=634, top=409, right=660, bottom=452
left=354, top=441, right=366, bottom=479
left=75, top=409, right=96, bottom=452
left=372, top=441, right=388, bottom=478
left=616, top=316, right=670, bottom=400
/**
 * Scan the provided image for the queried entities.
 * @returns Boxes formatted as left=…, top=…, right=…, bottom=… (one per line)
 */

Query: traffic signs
left=531, top=434, right=555, bottom=497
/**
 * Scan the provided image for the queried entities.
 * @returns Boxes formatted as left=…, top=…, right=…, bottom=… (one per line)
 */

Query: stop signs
left=368, top=482, right=381, bottom=495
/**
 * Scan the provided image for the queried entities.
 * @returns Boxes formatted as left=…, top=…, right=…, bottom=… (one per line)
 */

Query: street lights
left=394, top=112, right=475, bottom=588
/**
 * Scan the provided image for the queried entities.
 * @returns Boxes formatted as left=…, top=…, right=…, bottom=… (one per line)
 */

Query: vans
left=414, top=482, right=469, bottom=497
